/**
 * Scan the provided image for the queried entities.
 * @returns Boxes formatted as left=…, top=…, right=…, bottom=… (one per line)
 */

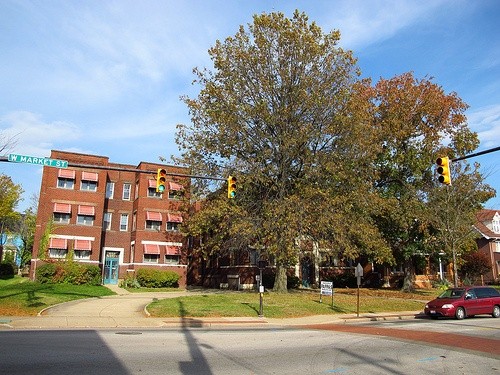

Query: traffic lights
left=228, top=176, right=237, bottom=199
left=156, top=168, right=167, bottom=193
left=436, top=156, right=451, bottom=185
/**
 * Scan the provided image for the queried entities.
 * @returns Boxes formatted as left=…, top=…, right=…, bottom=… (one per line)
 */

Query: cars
left=423, top=287, right=500, bottom=321
